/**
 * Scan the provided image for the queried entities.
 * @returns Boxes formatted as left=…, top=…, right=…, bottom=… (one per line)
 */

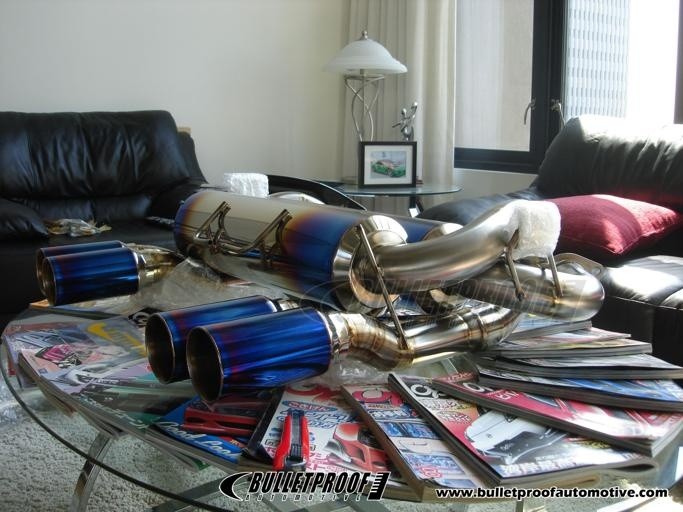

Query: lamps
left=329, top=28, right=408, bottom=184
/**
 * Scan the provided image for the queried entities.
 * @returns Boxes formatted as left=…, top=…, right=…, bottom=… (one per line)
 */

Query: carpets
left=0, top=343, right=683, bottom=511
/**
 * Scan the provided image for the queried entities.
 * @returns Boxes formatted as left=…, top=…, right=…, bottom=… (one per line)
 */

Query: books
left=3, top=314, right=683, bottom=506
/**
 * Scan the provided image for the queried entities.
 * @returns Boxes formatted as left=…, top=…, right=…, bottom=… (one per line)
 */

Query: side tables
left=326, top=181, right=462, bottom=217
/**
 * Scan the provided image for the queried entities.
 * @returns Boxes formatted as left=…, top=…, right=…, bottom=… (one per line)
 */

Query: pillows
left=542, top=187, right=683, bottom=260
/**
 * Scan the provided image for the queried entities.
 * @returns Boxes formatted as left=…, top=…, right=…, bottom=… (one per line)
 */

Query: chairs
left=176, top=125, right=368, bottom=212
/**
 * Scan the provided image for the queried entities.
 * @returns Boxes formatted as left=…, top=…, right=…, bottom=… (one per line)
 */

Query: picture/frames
left=358, top=142, right=416, bottom=189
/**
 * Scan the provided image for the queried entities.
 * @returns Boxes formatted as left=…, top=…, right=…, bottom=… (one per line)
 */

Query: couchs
left=415, top=114, right=683, bottom=368
left=0, top=110, right=209, bottom=332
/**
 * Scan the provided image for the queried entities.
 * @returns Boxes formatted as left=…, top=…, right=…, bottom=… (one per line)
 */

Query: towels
left=217, top=172, right=269, bottom=199
